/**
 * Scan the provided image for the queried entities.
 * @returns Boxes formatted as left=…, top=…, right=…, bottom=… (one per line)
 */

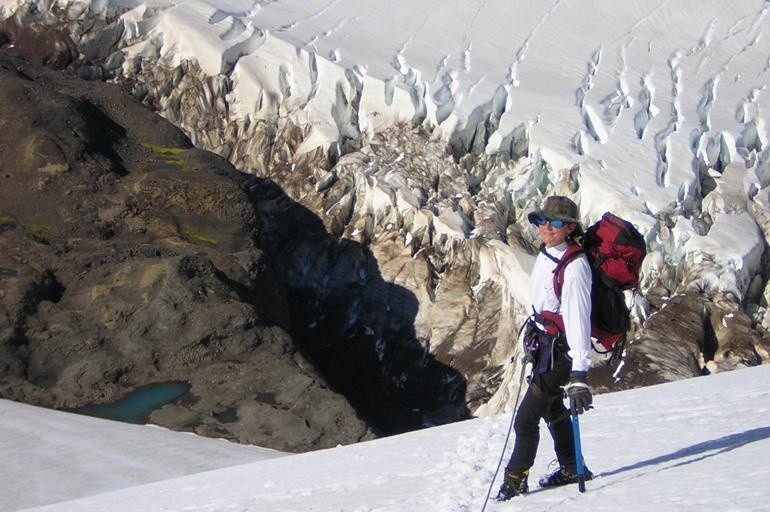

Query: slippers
left=552, top=211, right=647, bottom=344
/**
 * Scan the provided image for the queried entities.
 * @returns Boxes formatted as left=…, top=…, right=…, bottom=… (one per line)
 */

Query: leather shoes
left=568, top=387, right=593, bottom=415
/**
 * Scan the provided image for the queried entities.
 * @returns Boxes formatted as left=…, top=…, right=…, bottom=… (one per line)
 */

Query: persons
left=492, top=196, right=592, bottom=502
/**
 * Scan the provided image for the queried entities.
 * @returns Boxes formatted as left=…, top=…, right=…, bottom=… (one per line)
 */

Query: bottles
left=528, top=196, right=583, bottom=236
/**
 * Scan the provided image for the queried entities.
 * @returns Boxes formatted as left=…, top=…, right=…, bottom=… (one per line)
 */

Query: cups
left=536, top=214, right=565, bottom=228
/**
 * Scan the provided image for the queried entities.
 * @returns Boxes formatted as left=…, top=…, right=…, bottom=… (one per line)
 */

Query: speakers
left=497, top=467, right=530, bottom=499
left=539, top=456, right=593, bottom=486
